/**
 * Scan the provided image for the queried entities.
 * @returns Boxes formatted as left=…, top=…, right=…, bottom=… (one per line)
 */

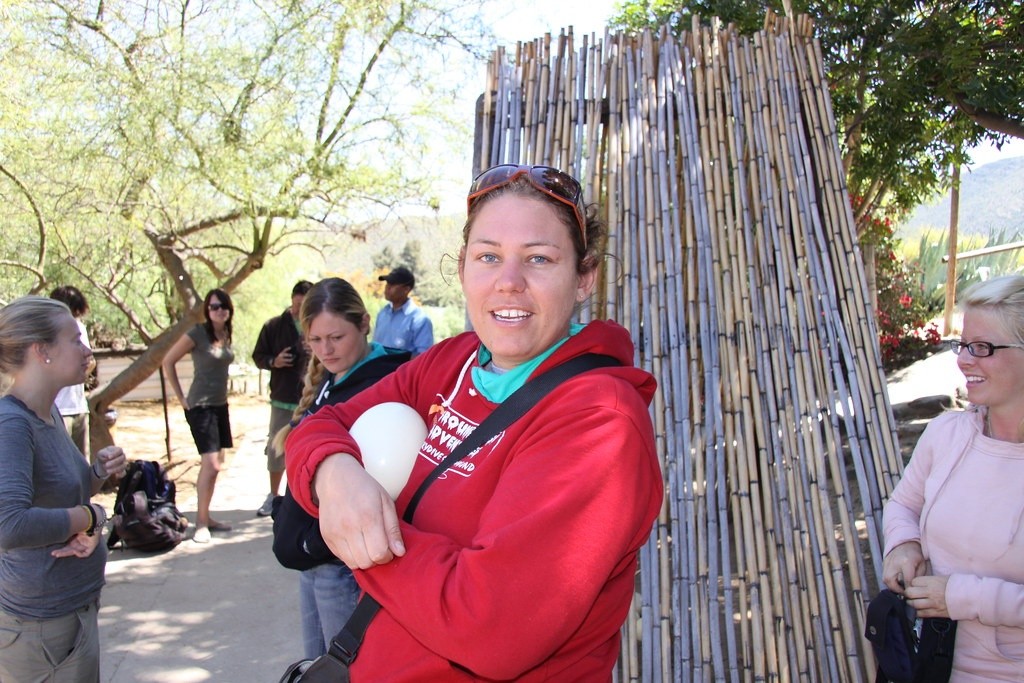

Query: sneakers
left=257, top=493, right=277, bottom=517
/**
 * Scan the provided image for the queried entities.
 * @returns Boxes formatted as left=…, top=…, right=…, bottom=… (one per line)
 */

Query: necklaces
left=985, top=410, right=994, bottom=438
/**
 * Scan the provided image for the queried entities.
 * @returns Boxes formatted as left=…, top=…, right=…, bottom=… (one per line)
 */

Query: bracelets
left=93, top=504, right=106, bottom=526
left=83, top=504, right=92, bottom=532
left=85, top=504, right=97, bottom=537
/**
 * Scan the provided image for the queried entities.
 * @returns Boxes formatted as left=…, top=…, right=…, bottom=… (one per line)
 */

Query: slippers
left=210, top=523, right=234, bottom=532
left=192, top=528, right=211, bottom=544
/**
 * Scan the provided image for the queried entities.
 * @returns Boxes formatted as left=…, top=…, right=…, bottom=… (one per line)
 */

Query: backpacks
left=104, top=459, right=189, bottom=556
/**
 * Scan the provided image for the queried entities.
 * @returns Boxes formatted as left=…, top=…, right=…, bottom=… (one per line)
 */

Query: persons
left=270, top=278, right=396, bottom=658
left=288, top=162, right=663, bottom=682
left=880, top=269, right=1024, bottom=683
left=252, top=278, right=320, bottom=517
left=370, top=265, right=435, bottom=353
left=162, top=287, right=235, bottom=543
left=1, top=282, right=131, bottom=683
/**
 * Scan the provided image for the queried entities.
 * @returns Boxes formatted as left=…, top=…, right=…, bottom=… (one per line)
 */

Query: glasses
left=464, top=162, right=589, bottom=251
left=949, top=339, right=1024, bottom=358
left=206, top=303, right=227, bottom=311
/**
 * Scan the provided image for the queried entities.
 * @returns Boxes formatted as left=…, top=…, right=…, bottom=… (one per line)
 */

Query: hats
left=378, top=266, right=416, bottom=289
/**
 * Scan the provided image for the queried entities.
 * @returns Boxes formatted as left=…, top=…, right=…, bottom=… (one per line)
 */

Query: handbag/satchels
left=275, top=650, right=353, bottom=683
left=864, top=589, right=959, bottom=683
